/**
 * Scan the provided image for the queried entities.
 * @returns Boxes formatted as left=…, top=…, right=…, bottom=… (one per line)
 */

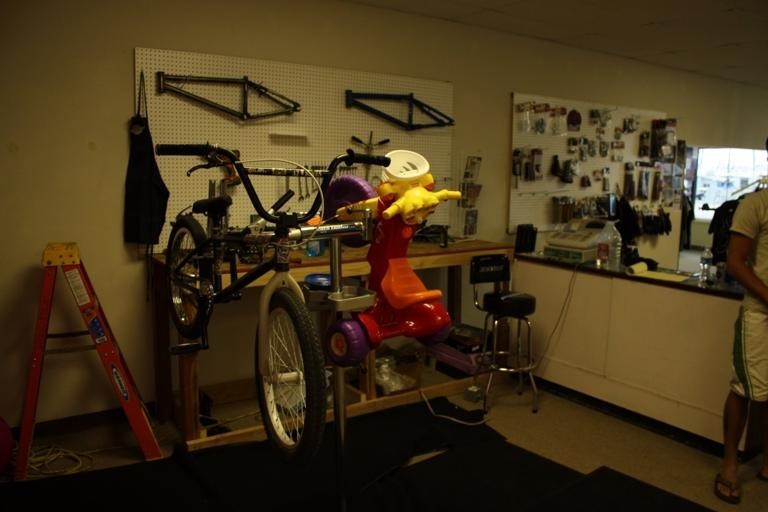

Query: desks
left=140, top=237, right=523, bottom=460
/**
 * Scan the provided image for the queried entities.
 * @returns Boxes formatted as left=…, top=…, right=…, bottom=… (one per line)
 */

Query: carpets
left=0, top=396, right=718, bottom=512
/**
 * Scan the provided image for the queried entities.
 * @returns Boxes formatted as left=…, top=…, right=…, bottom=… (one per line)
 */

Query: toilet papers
left=625, top=262, right=689, bottom=283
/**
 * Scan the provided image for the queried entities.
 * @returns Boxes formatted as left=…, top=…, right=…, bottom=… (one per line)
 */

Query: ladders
left=16, top=241, right=163, bottom=479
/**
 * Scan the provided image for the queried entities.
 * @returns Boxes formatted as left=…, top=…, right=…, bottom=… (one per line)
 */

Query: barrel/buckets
left=596, top=219, right=622, bottom=271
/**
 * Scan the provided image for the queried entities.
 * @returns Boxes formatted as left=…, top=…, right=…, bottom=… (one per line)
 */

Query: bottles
left=699, top=246, right=712, bottom=286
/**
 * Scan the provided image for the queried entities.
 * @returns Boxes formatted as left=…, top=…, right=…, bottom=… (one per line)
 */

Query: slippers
left=714, top=471, right=741, bottom=504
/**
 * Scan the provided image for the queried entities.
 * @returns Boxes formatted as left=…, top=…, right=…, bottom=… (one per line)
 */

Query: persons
left=717, top=139, right=768, bottom=496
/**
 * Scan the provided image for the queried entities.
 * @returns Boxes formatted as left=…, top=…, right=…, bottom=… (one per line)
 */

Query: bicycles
left=156, top=141, right=391, bottom=466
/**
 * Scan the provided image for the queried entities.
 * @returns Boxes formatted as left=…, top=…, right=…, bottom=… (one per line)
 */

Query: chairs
left=467, top=254, right=542, bottom=417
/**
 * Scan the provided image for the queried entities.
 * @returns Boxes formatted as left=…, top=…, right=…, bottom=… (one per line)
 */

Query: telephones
left=548, top=218, right=622, bottom=249
